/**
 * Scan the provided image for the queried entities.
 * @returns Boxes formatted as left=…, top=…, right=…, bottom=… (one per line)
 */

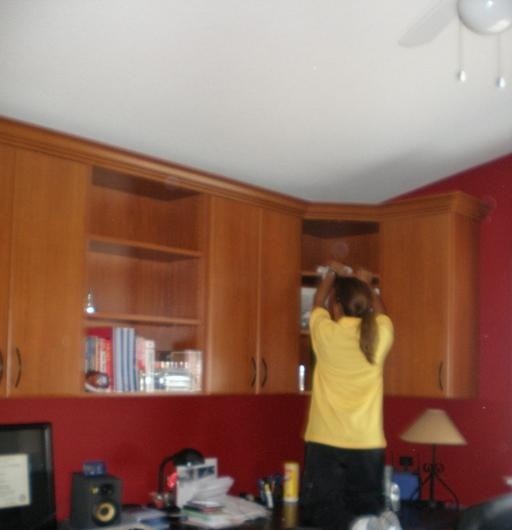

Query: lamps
left=148, top=447, right=203, bottom=513
left=397, top=408, right=467, bottom=496
left=457, top=0, right=512, bottom=88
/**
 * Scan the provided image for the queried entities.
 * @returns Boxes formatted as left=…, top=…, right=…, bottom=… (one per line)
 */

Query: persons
left=298, top=257, right=395, bottom=508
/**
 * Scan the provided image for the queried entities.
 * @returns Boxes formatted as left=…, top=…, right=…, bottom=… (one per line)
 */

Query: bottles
left=382, top=448, right=402, bottom=512
left=282, top=462, right=300, bottom=503
left=297, top=363, right=305, bottom=392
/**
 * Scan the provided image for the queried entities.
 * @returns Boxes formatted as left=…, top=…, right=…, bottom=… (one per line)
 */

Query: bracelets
left=328, top=268, right=338, bottom=277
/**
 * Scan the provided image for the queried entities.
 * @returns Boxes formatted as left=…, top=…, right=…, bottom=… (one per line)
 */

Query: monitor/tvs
left=0, top=423, right=56, bottom=529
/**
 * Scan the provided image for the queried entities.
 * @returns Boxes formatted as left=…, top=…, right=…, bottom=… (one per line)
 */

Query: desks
left=134, top=498, right=466, bottom=530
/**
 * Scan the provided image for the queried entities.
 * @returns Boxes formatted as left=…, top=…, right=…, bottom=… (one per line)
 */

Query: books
left=84, top=324, right=204, bottom=393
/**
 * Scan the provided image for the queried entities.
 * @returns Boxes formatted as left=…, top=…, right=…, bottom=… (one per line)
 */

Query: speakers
left=72, top=472, right=123, bottom=528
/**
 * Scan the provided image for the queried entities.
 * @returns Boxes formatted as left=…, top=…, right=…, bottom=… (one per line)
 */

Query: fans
left=397, top=0, right=456, bottom=47
